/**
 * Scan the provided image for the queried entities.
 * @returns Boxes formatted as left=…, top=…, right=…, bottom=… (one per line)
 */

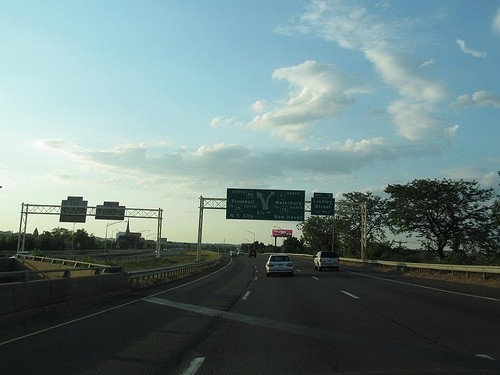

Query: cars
left=230, top=248, right=244, bottom=257
left=312, top=250, right=341, bottom=272
left=265, top=253, right=295, bottom=277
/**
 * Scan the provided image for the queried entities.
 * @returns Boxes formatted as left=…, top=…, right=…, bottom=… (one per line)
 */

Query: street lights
left=245, top=230, right=255, bottom=243
left=135, top=229, right=150, bottom=248
left=274, top=226, right=285, bottom=253
left=105, top=220, right=127, bottom=249
